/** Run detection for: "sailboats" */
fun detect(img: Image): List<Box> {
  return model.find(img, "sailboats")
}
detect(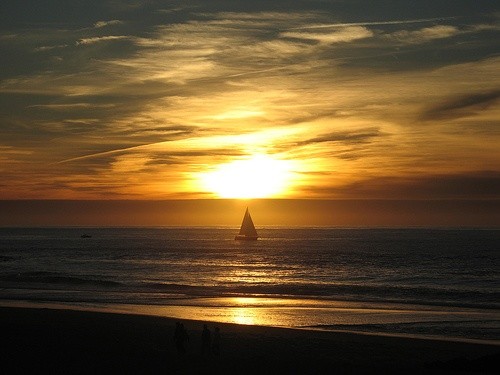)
[235,205,258,240]
